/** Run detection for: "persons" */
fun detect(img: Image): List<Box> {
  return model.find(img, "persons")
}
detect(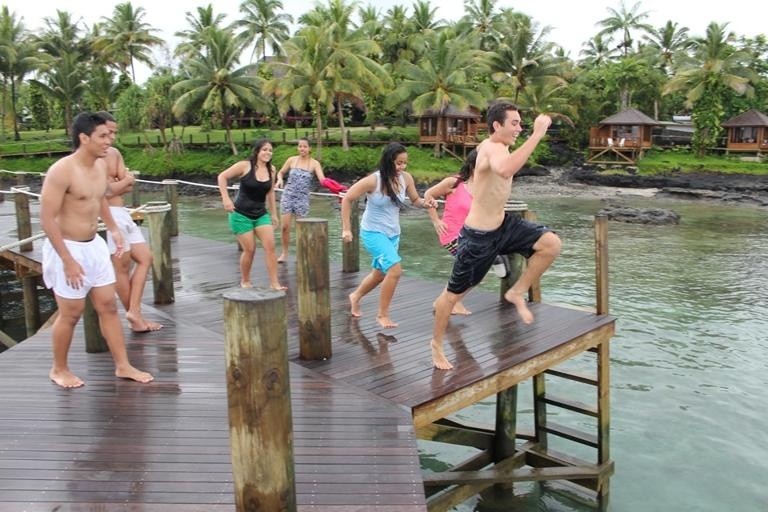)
[275,137,327,263]
[96,110,164,332]
[216,140,290,294]
[40,107,155,387]
[429,103,566,370]
[423,147,477,316]
[341,140,439,330]
